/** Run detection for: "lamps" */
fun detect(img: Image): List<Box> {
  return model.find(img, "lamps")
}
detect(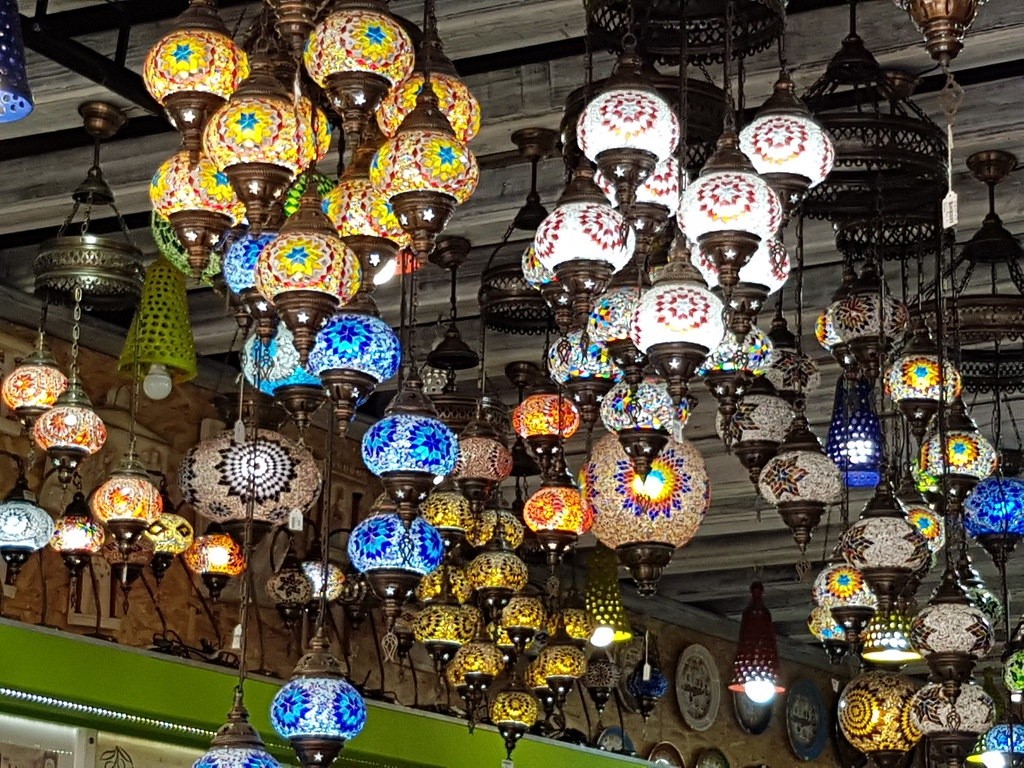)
[0,0,1024,768]
